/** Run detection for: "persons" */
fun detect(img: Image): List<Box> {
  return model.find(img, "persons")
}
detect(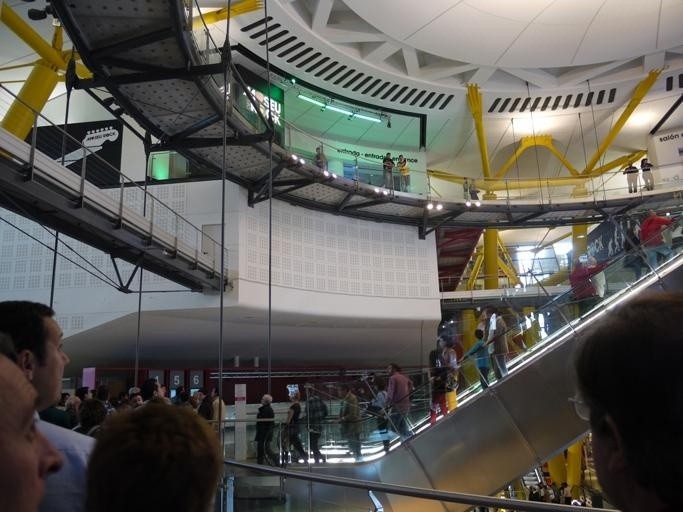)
[469,184,480,200]
[315,147,327,170]
[429,306,508,420]
[641,158,654,191]
[336,382,363,461]
[372,364,413,452]
[383,153,395,189]
[0,330,64,511]
[0,300,100,511]
[86,401,224,512]
[623,212,674,281]
[576,289,683,512]
[252,385,329,467]
[39,380,225,439]
[570,256,606,316]
[397,155,409,192]
[528,481,572,506]
[623,161,639,193]
